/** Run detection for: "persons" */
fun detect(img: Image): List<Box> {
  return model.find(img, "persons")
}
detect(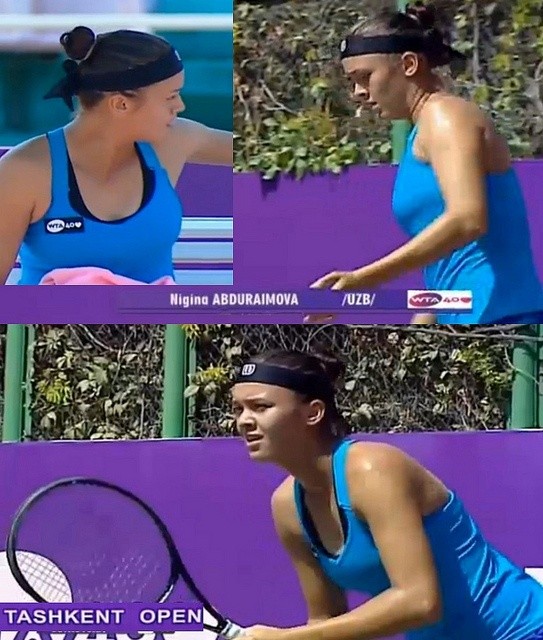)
[231,349,542,640]
[0,26,233,287]
[309,9,543,325]
